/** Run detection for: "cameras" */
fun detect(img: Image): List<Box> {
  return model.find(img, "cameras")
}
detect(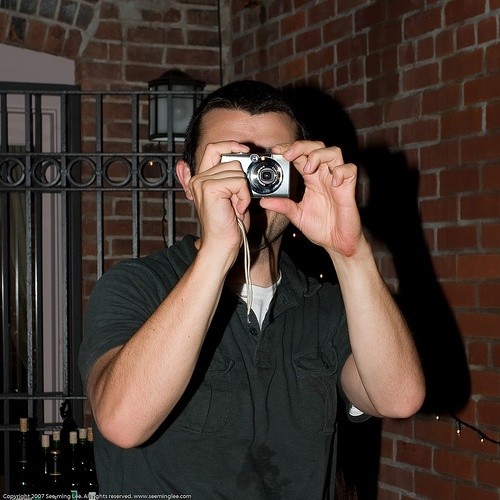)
[221,152,291,199]
